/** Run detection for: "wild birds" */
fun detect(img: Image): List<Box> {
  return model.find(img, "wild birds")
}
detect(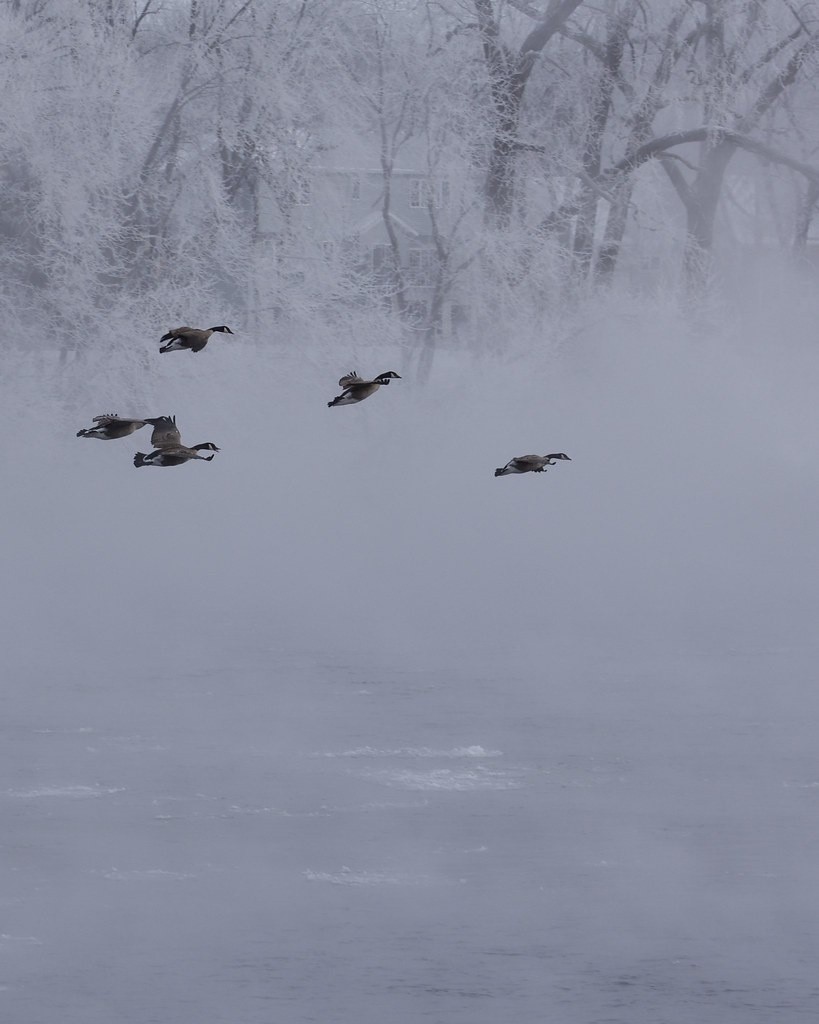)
[159,325,236,354]
[76,413,223,469]
[326,370,403,408]
[494,453,573,478]
